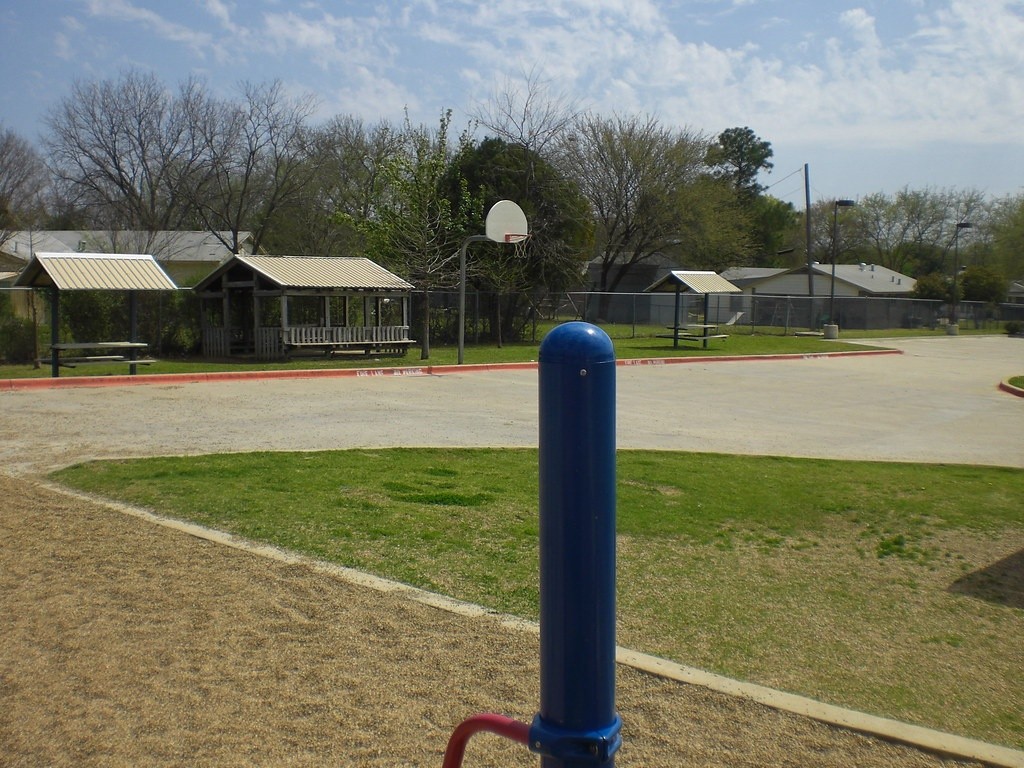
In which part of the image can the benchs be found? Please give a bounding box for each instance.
[684,335,729,340]
[65,360,156,367]
[34,355,123,361]
[655,333,690,336]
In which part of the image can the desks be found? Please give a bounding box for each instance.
[666,325,719,329]
[46,342,148,349]
